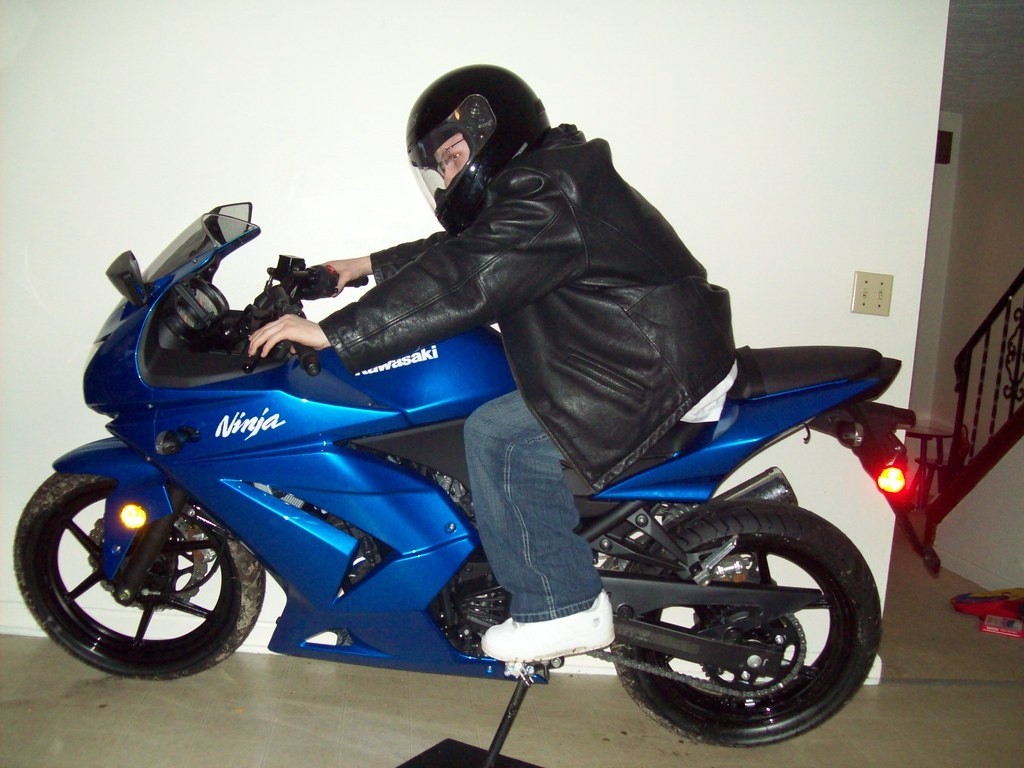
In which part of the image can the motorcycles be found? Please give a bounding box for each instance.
[13,200,915,768]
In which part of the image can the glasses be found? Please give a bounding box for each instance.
[435,138,464,179]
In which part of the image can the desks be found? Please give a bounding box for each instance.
[906,427,954,511]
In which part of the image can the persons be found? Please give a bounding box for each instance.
[248,66,739,664]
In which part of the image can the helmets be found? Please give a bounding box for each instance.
[406,65,550,237]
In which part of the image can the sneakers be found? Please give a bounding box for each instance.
[480,587,616,662]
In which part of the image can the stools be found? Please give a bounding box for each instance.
[913,458,947,504]
